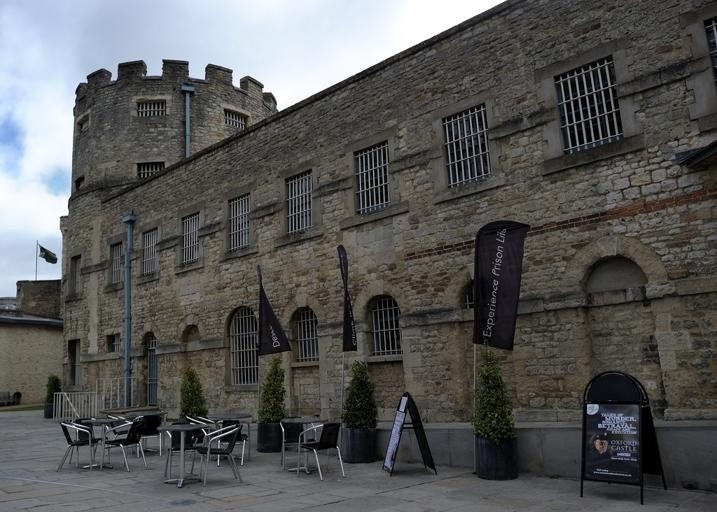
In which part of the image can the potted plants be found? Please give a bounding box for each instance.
[253,354,290,453]
[169,368,208,451]
[467,345,521,481]
[334,357,381,464]
[42,374,63,420]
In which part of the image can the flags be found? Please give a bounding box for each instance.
[38,246,57,264]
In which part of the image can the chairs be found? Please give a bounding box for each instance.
[53,404,349,489]
[0,391,21,406]
